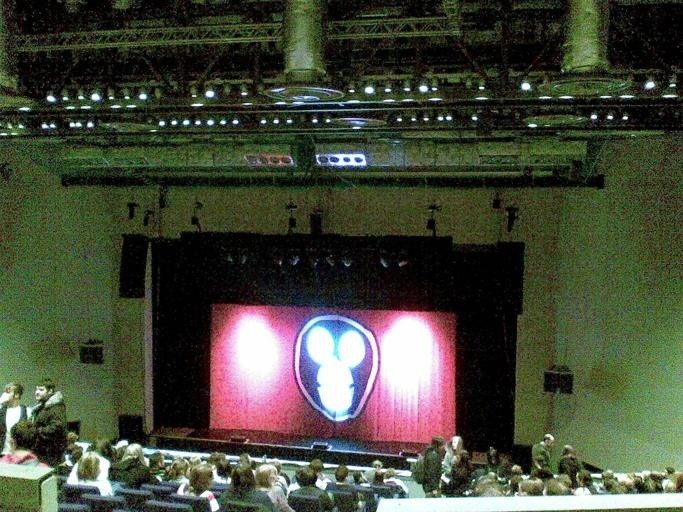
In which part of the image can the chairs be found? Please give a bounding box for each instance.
[1,435,681,512]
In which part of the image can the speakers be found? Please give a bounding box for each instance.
[80,343,103,363]
[116,232,149,297]
[119,414,144,442]
[543,371,573,393]
[509,444,533,473]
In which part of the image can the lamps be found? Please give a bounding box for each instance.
[1,68,683,136]
[125,184,519,277]
